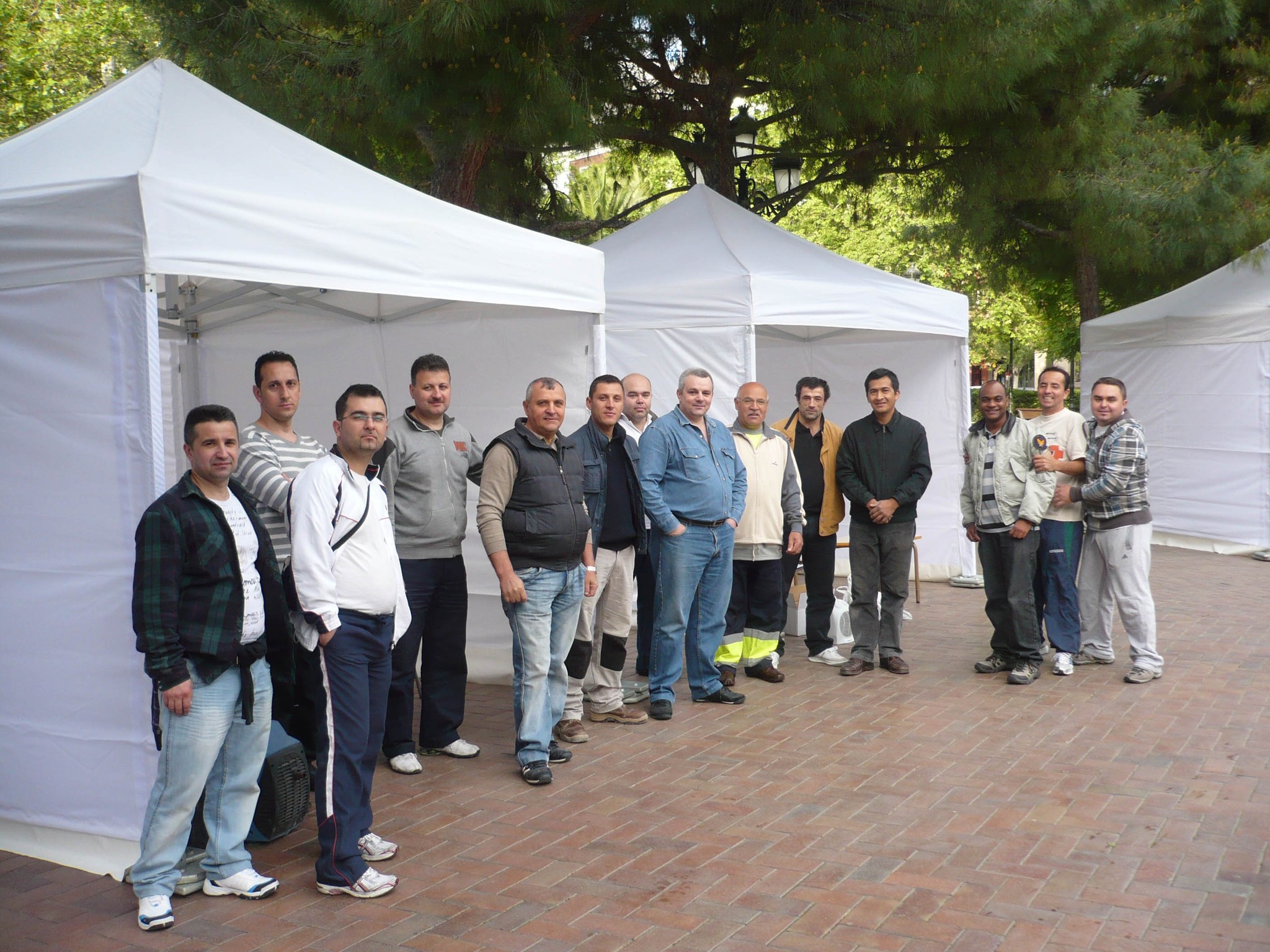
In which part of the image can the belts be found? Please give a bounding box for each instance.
[672,514,727,527]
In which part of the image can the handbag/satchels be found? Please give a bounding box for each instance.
[836,575,852,605]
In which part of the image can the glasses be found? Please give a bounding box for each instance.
[340,413,389,424]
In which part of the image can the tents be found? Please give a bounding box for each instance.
[2,56,608,883]
[1079,236,1270,560]
[590,168,986,589]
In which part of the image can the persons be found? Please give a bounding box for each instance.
[964,366,1082,675]
[834,368,932,676]
[713,381,808,689]
[372,352,487,774]
[127,405,280,932]
[617,373,656,677]
[478,377,598,784]
[638,366,747,720]
[960,380,1058,683]
[283,385,418,899]
[1052,377,1164,684]
[553,375,647,743]
[767,377,850,670]
[229,350,330,789]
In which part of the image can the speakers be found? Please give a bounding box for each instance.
[248,720,315,843]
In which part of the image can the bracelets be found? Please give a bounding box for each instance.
[585,566,597,574]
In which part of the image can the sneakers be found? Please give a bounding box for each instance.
[317,866,399,898]
[649,700,672,719]
[718,670,736,687]
[137,893,174,931]
[879,654,910,674]
[746,665,786,683]
[1124,666,1163,682]
[390,753,422,774]
[421,738,480,757]
[555,717,589,743]
[357,833,399,861]
[1007,659,1040,685]
[975,656,1013,673]
[1072,651,1115,665]
[521,760,553,784]
[548,740,572,763]
[840,659,874,676]
[202,866,281,900]
[1038,643,1049,656]
[695,685,746,704]
[590,704,647,724]
[1051,651,1073,676]
[808,646,848,666]
